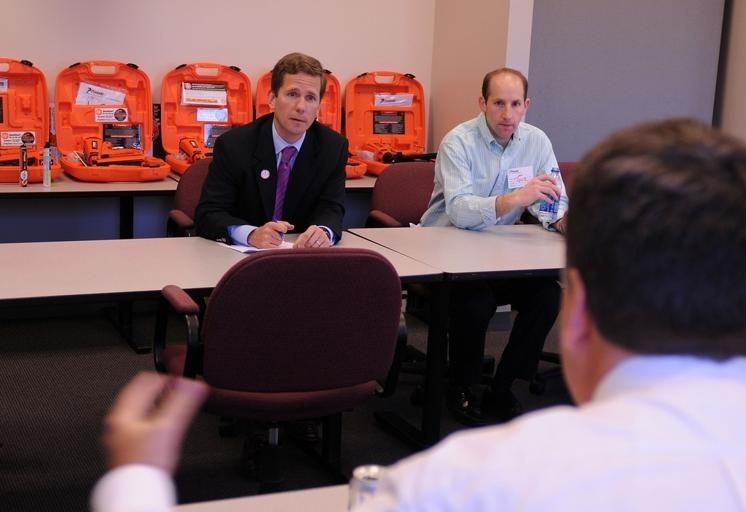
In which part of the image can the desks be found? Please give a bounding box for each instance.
[168,174,342,189]
[348,224,566,451]
[0,175,179,353]
[0,231,444,484]
[344,174,375,189]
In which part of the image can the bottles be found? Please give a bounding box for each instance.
[42,142,52,190]
[20,144,28,187]
[538,167,563,223]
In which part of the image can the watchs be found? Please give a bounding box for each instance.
[320,227,331,241]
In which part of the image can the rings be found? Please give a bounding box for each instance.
[316,241,321,247]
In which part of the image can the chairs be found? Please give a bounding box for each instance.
[151,247,408,496]
[164,156,213,237]
[369,162,545,420]
[558,160,577,197]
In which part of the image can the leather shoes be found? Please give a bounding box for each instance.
[484,385,521,416]
[447,386,475,423]
[293,420,322,461]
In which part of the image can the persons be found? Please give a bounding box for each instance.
[409,68,569,237]
[193,51,349,249]
[85,117,745,512]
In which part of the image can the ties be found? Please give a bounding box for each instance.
[275,147,296,237]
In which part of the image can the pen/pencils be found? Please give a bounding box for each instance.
[273,217,285,239]
